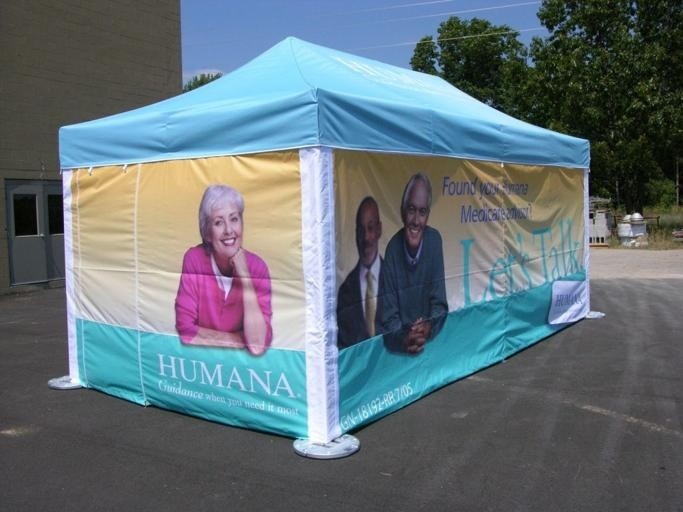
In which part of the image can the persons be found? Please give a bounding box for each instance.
[174,184,273,357]
[376,173,449,354]
[335,196,386,348]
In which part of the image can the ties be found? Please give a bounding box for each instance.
[363,271,378,337]
